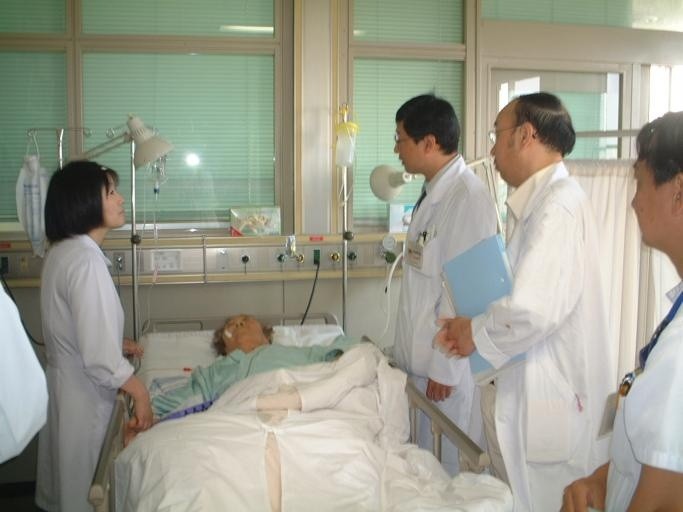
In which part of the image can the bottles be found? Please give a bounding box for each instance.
[336,121,358,166]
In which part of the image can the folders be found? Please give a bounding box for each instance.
[440,234,530,386]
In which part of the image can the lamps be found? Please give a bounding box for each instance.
[65,111,172,171]
[369,153,506,235]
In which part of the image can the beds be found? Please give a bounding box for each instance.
[88,310,491,512]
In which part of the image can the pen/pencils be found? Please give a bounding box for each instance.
[418,225,434,242]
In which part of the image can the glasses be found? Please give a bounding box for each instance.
[394,133,414,145]
[487,127,517,146]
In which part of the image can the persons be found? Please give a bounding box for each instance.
[120,302,381,449]
[387,89,504,479]
[0,278,51,465]
[30,159,153,511]
[556,106,683,512]
[430,91,623,512]
